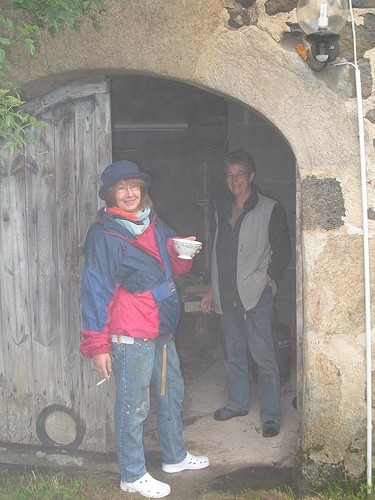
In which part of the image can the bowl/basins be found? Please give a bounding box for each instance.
[172,239,202,260]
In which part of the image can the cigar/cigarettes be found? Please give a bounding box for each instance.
[97,375,111,387]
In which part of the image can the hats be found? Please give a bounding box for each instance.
[98,160,151,200]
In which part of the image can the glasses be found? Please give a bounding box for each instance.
[116,184,140,192]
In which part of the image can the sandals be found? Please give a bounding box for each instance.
[213,407,247,420]
[262,421,279,436]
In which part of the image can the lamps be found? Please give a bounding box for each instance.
[296,0,348,73]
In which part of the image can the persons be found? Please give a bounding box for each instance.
[201,149,290,437]
[82,158,212,498]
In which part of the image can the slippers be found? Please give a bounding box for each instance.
[161,452,210,475]
[119,472,171,498]
